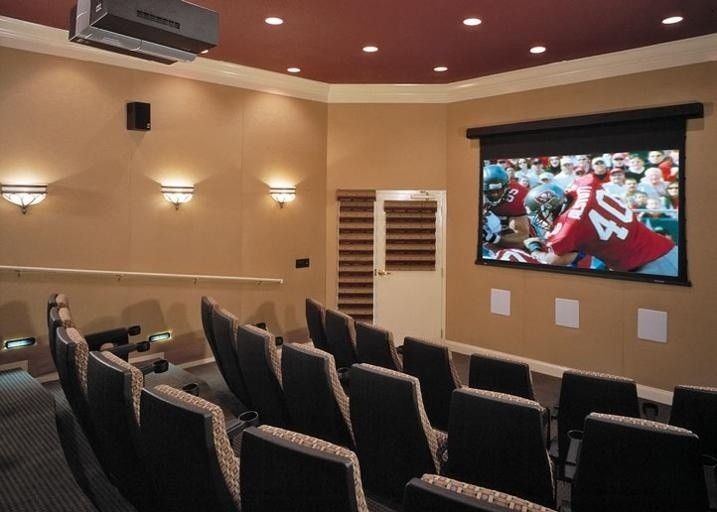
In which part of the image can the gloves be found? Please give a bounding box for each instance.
[524,237,541,252]
[482,223,496,243]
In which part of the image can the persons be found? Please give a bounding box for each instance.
[481,149,680,277]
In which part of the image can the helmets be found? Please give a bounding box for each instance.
[523,182,567,241]
[483,164,510,207]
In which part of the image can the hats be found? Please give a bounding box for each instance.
[560,157,572,165]
[592,158,604,166]
[612,153,625,160]
[609,168,624,174]
[533,159,543,164]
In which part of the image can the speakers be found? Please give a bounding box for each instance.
[127,101,151,131]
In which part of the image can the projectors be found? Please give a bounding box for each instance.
[68,0,221,66]
